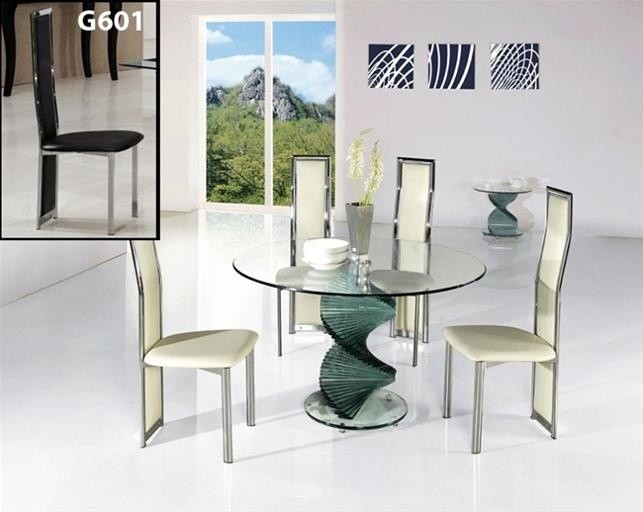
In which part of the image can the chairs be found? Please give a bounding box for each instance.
[277,154,338,357]
[129,239,258,464]
[390,156,435,367]
[33,8,145,235]
[442,186,573,453]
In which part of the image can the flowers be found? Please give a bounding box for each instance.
[346,126,381,205]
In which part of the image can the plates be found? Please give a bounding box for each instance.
[301,237,352,272]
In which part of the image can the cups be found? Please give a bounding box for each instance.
[355,259,372,292]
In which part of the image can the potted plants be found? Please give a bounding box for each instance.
[345,203,374,254]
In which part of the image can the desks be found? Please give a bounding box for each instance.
[474,184,533,238]
